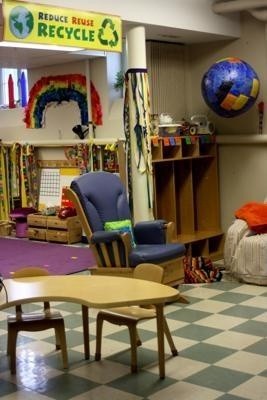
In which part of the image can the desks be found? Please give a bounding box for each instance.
[0,275,181,379]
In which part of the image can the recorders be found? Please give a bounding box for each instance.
[189,114,216,136]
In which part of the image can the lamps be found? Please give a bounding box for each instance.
[71,124,96,140]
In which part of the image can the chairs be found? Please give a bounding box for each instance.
[64,171,185,287]
[95,263,177,373]
[8,268,69,375]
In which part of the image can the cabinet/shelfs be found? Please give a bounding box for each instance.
[150,136,225,262]
[27,212,82,245]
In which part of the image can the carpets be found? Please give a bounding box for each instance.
[0,236,97,280]
